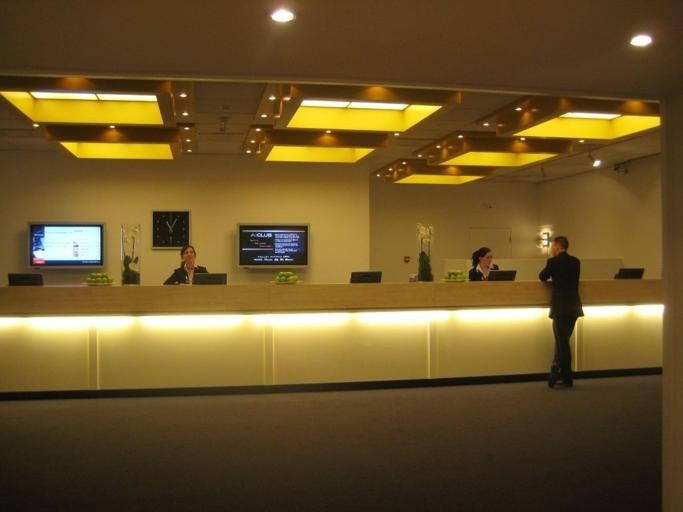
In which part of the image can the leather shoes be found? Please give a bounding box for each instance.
[558,381,572,387]
[549,365,560,388]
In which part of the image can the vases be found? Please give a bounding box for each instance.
[417,251,433,281]
[120,262,140,286]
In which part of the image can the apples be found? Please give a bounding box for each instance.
[86,273,113,283]
[444,269,466,279]
[276,272,299,282]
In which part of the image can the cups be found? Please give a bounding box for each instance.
[408,273,418,281]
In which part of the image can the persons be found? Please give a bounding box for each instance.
[538,236,584,387]
[163,244,209,285]
[469,247,499,281]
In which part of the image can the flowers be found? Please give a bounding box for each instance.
[121,221,141,266]
[414,221,434,257]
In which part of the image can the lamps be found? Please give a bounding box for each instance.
[474,94,663,139]
[409,129,579,168]
[367,155,497,186]
[0,73,196,130]
[44,121,198,161]
[253,81,462,134]
[537,231,551,248]
[242,123,389,166]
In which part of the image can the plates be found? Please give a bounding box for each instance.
[440,278,471,281]
[80,282,119,285]
[268,280,306,284]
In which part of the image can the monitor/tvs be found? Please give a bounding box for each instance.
[234,222,309,270]
[615,268,644,279]
[193,273,227,285]
[28,223,104,268]
[350,271,382,283]
[8,273,44,286]
[488,270,516,280]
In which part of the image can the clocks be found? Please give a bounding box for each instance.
[150,210,191,251]
